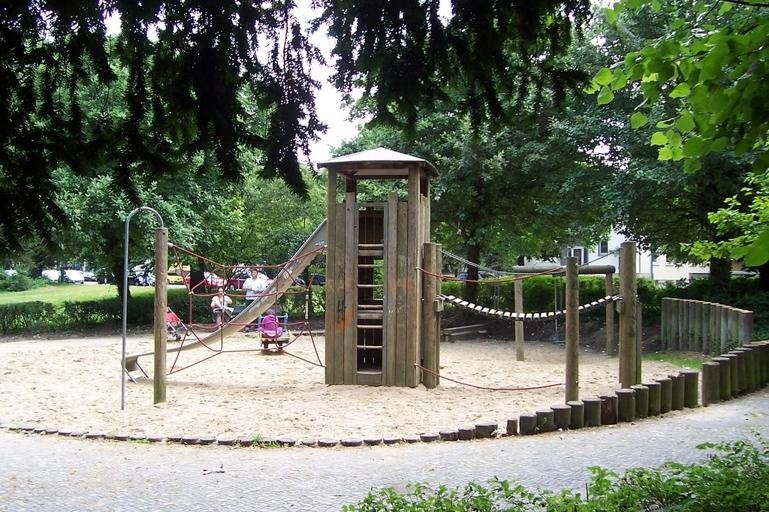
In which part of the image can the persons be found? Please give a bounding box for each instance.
[242,268,265,332]
[166,304,182,341]
[210,287,232,329]
[260,309,282,353]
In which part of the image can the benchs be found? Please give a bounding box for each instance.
[220,303,282,318]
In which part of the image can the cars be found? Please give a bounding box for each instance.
[3,267,115,285]
[125,257,326,291]
[455,271,483,283]
[441,272,455,281]
[480,272,496,278]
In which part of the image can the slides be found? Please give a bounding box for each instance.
[122,218,326,380]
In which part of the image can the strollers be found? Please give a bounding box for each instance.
[163,304,192,344]
